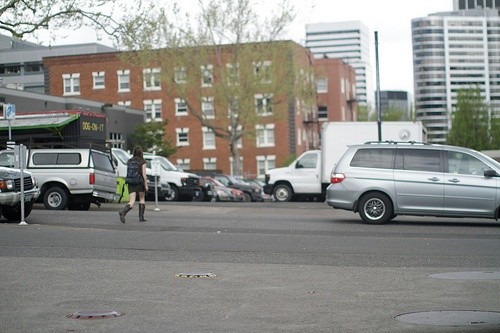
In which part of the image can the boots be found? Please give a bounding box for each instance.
[119,204,132,224]
[139,203,147,222]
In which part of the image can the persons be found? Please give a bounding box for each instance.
[118,146,148,224]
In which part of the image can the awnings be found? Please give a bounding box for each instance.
[0,113,80,130]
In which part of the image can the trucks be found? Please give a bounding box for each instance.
[263,122,427,201]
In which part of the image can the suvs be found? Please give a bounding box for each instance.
[0,166,40,223]
[130,155,201,201]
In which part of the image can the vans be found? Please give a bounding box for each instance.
[326,140,500,223]
[110,149,134,176]
[0,149,116,211]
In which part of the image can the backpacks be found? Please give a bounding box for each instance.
[124,159,146,185]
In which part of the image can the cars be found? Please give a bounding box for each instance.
[194,174,243,202]
[212,174,264,202]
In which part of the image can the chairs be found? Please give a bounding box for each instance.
[447,158,459,173]
